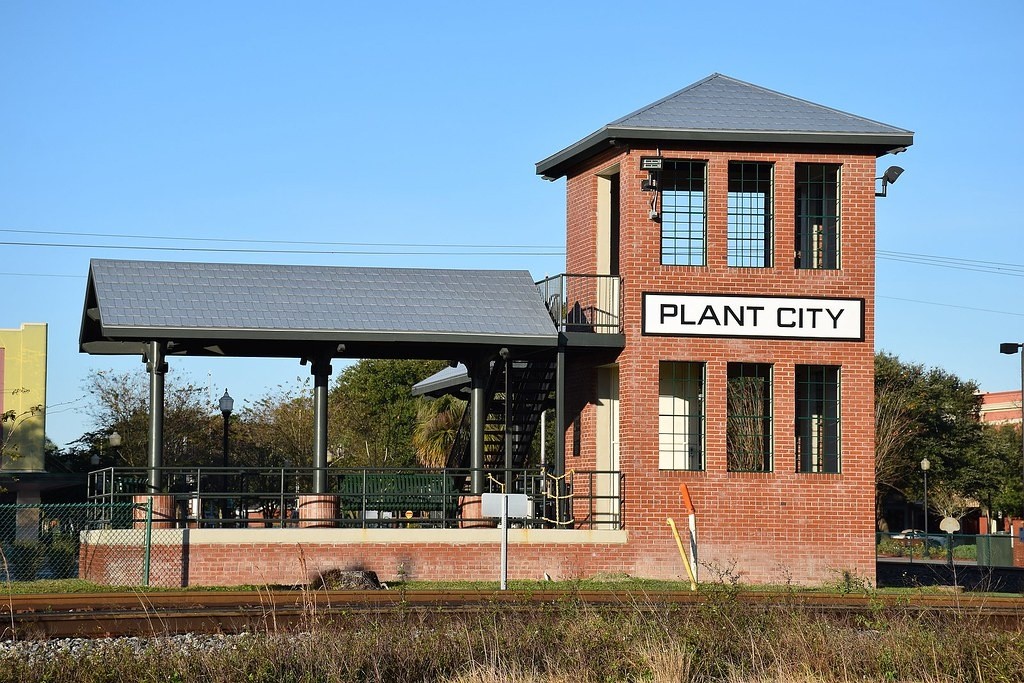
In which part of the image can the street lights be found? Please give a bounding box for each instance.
[219,387,234,528]
[110,432,121,502]
[91,454,99,471]
[920,456,931,556]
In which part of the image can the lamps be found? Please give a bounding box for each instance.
[639,155,663,191]
[875,166,905,197]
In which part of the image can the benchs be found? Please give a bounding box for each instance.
[336,472,460,528]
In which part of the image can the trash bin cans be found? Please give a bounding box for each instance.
[975,532,1014,566]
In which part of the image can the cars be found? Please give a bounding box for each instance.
[891,529,947,547]
